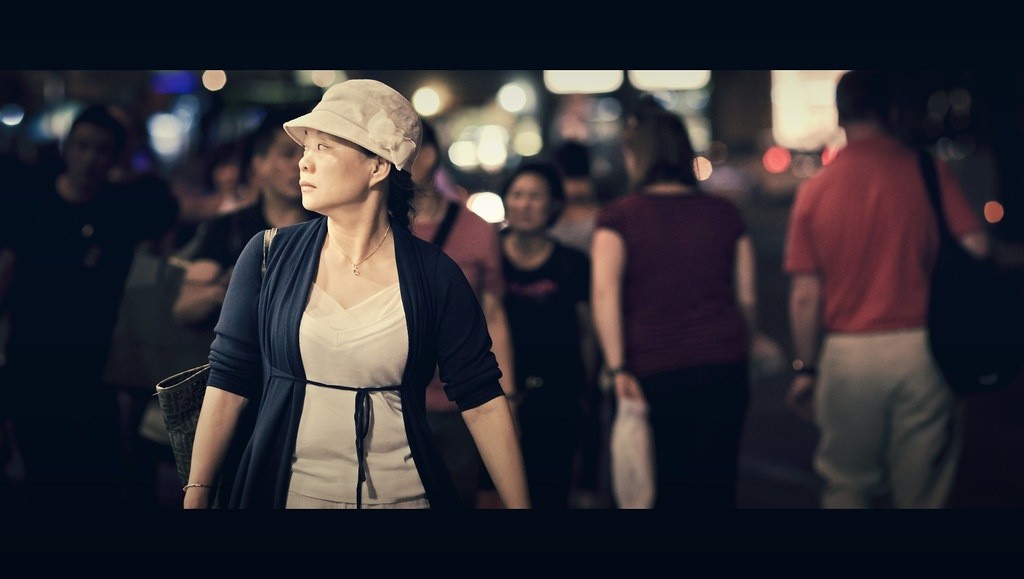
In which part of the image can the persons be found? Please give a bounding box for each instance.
[590,102,759,511]
[182,80,529,510]
[496,157,595,509]
[780,68,994,512]
[409,115,517,509]
[1,92,317,508]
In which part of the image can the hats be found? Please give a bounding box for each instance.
[283,79,425,175]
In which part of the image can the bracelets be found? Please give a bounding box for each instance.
[606,365,628,378]
[794,364,816,378]
[182,483,215,493]
[505,392,518,401]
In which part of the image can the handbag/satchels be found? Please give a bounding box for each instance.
[610,398,655,510]
[104,246,221,387]
[156,363,213,486]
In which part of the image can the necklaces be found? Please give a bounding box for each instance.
[325,223,391,276]
